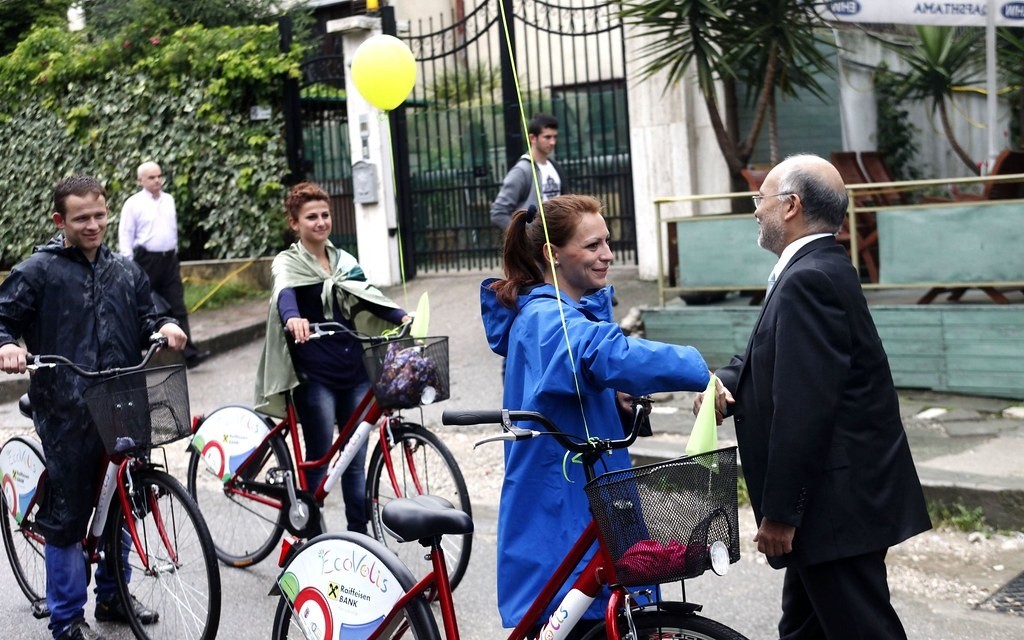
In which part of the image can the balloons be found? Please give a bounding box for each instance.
[351,34,418,110]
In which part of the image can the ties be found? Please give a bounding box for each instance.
[765,271,775,302]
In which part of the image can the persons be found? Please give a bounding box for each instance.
[271,183,414,537]
[479,193,710,640]
[115,161,214,370]
[0,174,189,640]
[489,113,568,233]
[691,150,937,640]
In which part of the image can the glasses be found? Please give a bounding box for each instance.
[752,191,796,209]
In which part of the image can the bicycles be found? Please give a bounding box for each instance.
[185,318,475,599]
[0,333,222,640]
[267,395,750,640]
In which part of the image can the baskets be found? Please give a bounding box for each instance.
[363,335,451,409]
[584,443,740,588]
[81,363,192,457]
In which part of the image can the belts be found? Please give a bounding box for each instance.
[147,249,177,257]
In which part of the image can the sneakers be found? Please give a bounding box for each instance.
[94,591,159,625]
[56,618,108,640]
